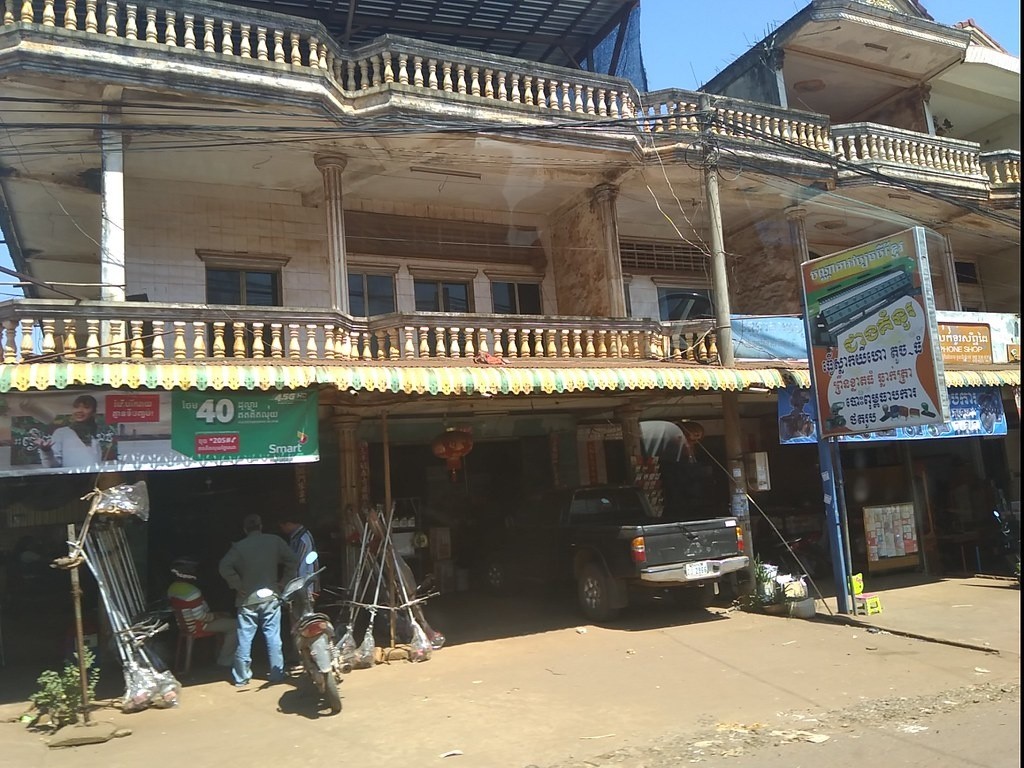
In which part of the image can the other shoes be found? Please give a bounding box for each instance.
[214,664,233,671]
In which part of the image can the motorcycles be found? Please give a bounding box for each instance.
[256,551,344,714]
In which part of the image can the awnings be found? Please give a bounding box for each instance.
[0,364,788,396]
[944,370,1021,388]
[780,369,812,390]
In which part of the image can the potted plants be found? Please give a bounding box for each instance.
[758,583,786,614]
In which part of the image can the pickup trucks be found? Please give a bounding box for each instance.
[484,482,750,620]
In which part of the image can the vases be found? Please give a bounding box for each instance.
[787,597,817,618]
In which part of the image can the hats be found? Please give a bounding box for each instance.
[243,513,262,528]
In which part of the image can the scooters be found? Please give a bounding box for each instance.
[992,510,1021,582]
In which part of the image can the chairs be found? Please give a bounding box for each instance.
[170,597,223,677]
[847,573,881,616]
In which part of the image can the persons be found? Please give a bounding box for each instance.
[32,395,102,468]
[219,513,299,687]
[275,511,320,673]
[167,555,238,669]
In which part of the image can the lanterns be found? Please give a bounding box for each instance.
[430,426,474,482]
[675,419,705,464]
